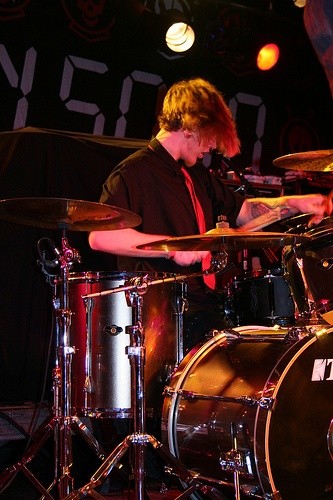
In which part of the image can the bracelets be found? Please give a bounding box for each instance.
[167,235,176,261]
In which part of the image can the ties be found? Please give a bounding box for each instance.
[181,165,216,291]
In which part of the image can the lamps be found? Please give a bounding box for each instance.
[156,8,197,55]
[245,27,279,71]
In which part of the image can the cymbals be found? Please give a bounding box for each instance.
[0,197,143,230]
[272,150,333,172]
[135,229,312,251]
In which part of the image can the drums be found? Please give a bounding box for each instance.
[159,324,333,500]
[53,267,189,422]
[223,266,310,325]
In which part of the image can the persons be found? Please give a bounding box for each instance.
[87,77,333,491]
